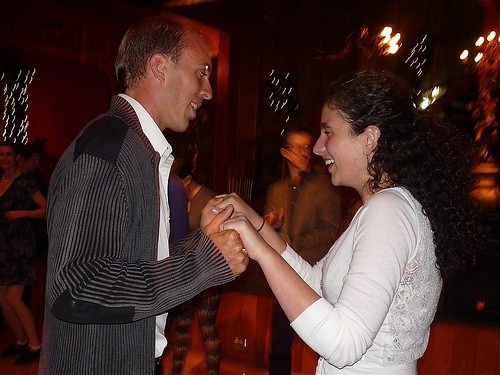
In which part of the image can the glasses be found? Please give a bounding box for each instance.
[285,144,312,150]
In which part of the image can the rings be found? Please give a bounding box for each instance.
[241,249,248,255]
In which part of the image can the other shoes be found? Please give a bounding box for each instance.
[0,341,27,360]
[14,347,42,366]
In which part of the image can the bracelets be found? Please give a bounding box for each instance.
[257,219,265,232]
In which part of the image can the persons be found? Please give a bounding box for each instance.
[38,17,250,375]
[263,131,342,375]
[169,164,222,375]
[213,70,491,374]
[0,139,49,365]
